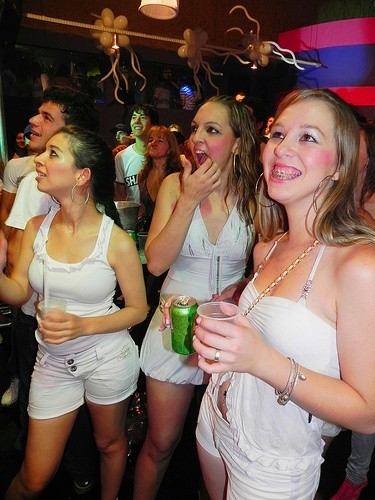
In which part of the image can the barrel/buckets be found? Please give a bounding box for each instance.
[95,201,142,250]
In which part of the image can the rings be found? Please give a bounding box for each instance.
[213,349,221,362]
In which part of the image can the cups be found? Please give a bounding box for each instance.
[137,232,148,256]
[37,297,67,341]
[197,300,241,366]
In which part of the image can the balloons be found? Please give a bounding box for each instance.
[176,27,209,70]
[88,7,130,56]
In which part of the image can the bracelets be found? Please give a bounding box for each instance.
[277,357,307,406]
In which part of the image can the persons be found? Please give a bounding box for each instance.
[31,65,204,110]
[134,94,284,500]
[235,97,275,195]
[0,85,99,452]
[330,124,375,500]
[158,87,375,500]
[241,31,273,68]
[0,123,149,500]
[107,103,196,250]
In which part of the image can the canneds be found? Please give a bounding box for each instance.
[169,295,200,355]
[126,229,137,248]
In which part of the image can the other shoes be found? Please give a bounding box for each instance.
[1,378,19,405]
[14,428,26,450]
[72,461,97,494]
[331,478,368,500]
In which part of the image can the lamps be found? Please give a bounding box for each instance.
[138,0,179,20]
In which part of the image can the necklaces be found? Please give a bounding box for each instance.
[209,189,234,213]
